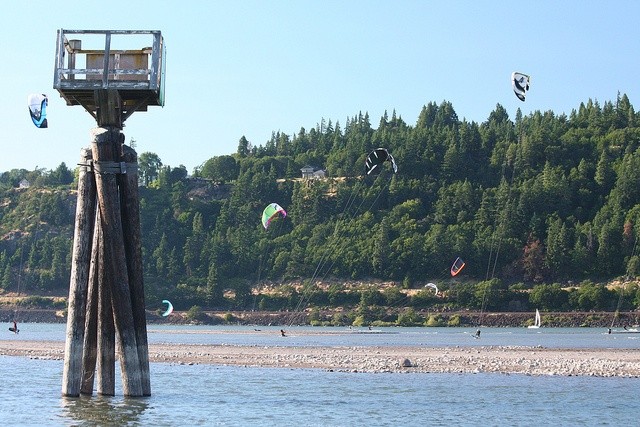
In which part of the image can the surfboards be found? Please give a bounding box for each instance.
[8,327,20,332]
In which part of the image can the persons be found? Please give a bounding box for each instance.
[369,324,372,330]
[623,320,629,330]
[605,328,618,334]
[472,329,481,339]
[281,328,286,336]
[13,320,17,333]
